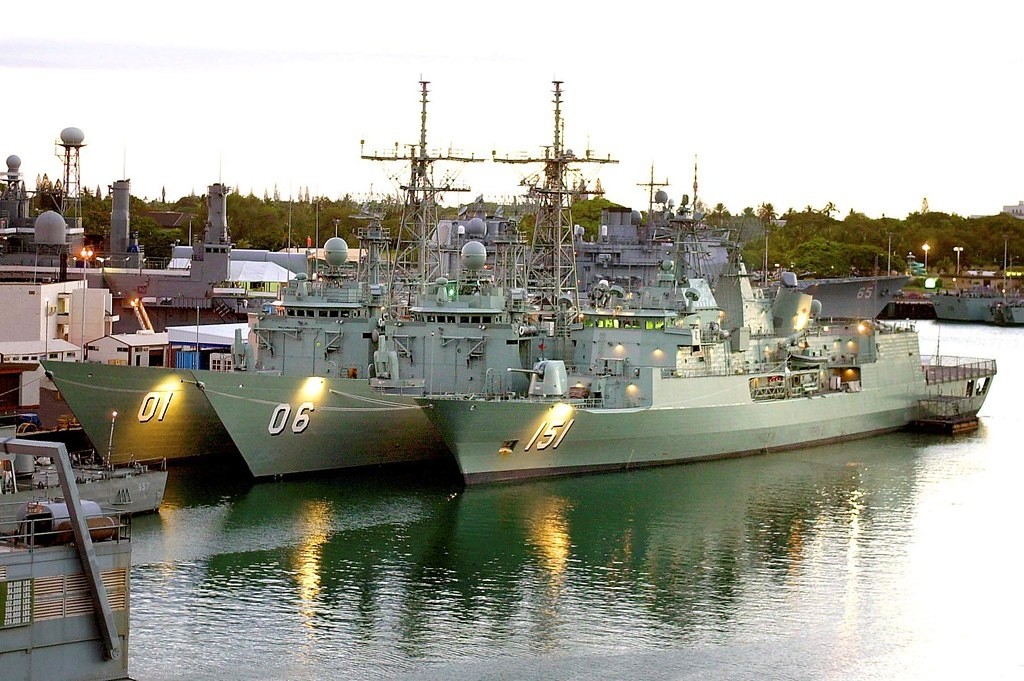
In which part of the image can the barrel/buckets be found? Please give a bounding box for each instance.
[340,368,349,378]
[349,368,357,379]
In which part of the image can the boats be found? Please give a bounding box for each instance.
[1,412,167,533]
[37,77,998,488]
[928,285,1024,327]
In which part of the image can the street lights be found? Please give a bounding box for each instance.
[922,245,930,270]
[954,247,963,277]
[80,250,93,363]
